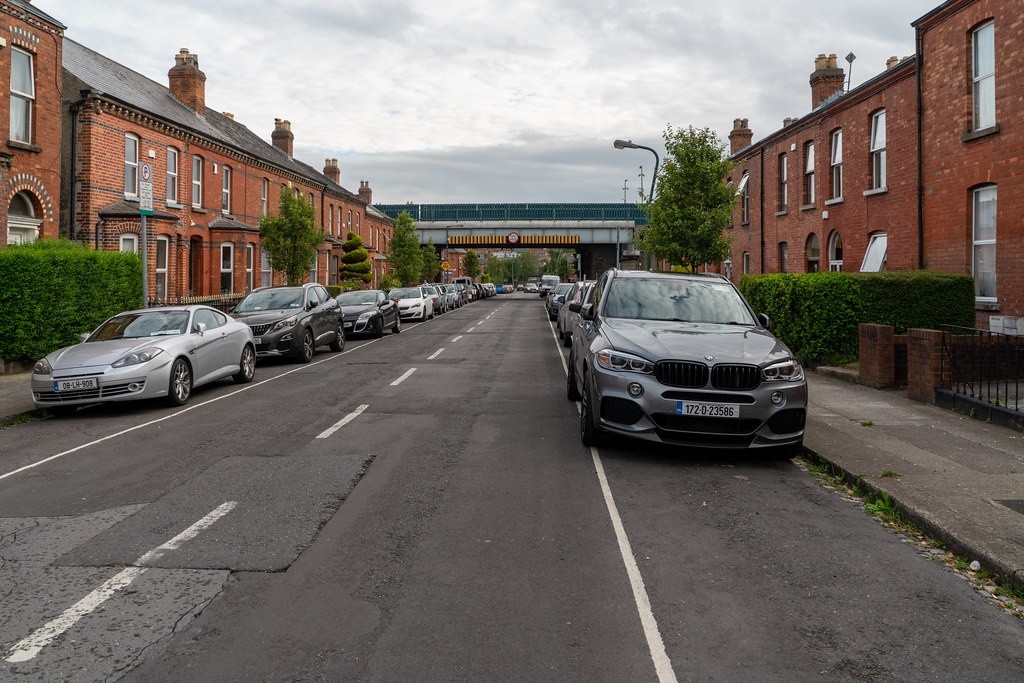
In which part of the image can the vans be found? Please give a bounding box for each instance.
[540,275,561,299]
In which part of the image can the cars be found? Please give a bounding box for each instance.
[335,289,402,338]
[385,287,434,322]
[30,305,258,417]
[525,282,540,293]
[505,287,513,293]
[419,277,498,315]
[547,283,578,321]
[496,284,506,294]
[557,279,597,347]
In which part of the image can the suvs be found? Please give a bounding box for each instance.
[226,282,345,363]
[566,267,809,460]
[517,284,525,291]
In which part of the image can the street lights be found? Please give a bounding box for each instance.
[512,261,515,283]
[613,139,660,203]
